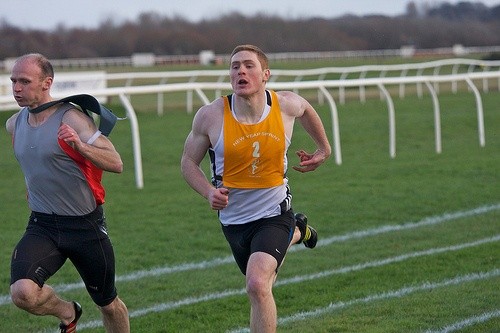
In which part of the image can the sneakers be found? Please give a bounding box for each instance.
[59,300,82,333]
[295,212,318,248]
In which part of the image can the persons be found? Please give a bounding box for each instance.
[6,54,139,332]
[181,45,332,332]
